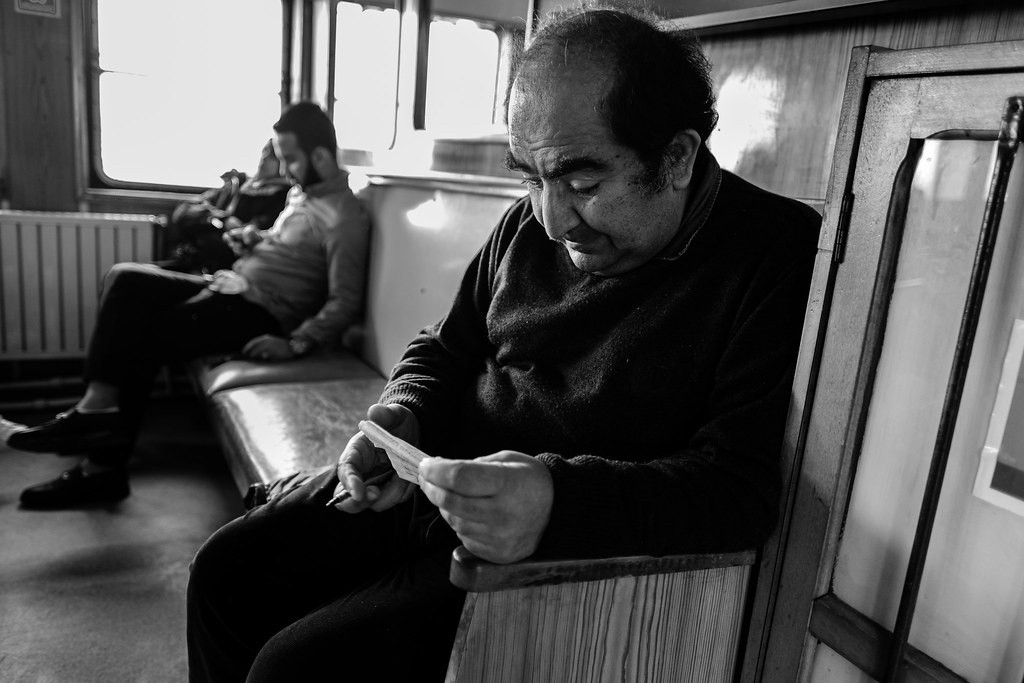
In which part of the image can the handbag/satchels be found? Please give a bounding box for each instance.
[172,176,240,224]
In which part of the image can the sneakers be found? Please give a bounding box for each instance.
[21,457,129,509]
[7,402,135,451]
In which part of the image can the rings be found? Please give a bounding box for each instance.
[262,351,268,358]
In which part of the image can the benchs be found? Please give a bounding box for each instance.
[183,173,759,683]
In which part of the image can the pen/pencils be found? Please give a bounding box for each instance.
[325,468,395,507]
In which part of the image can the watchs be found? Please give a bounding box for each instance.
[288,338,307,357]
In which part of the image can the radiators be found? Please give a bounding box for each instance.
[0,211,166,426]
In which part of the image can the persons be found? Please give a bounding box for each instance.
[7,101,370,513]
[188,7,822,682]
